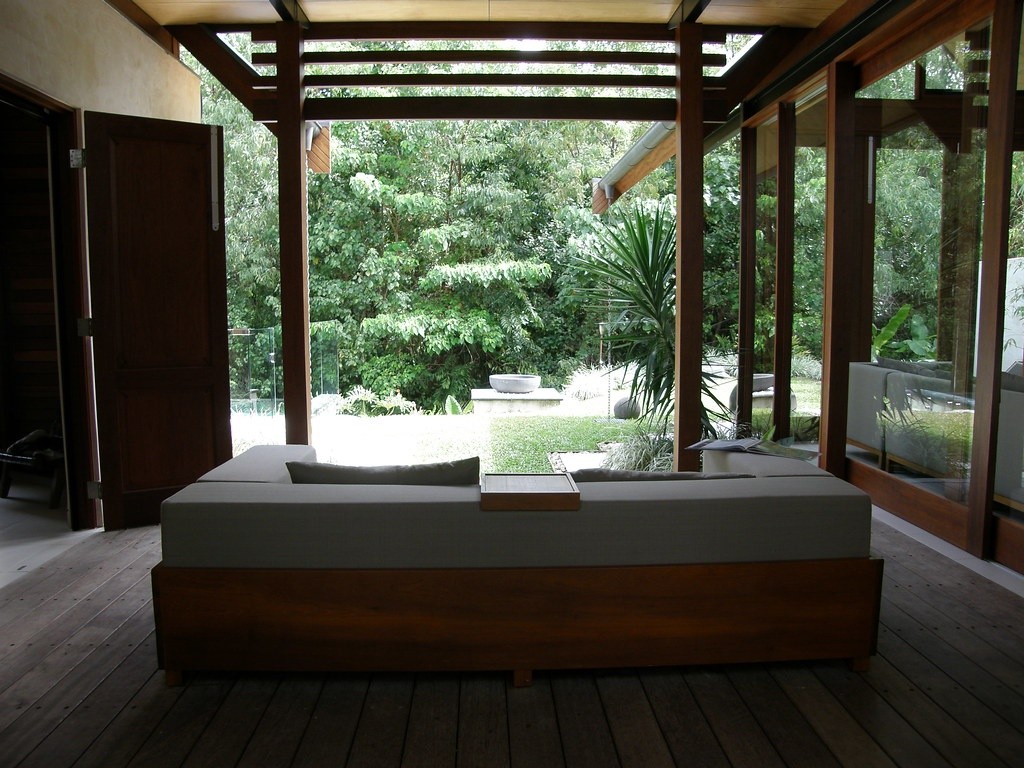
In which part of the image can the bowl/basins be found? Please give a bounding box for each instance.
[488,373,542,393]
[751,373,775,392]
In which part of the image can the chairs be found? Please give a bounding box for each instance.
[0,419,66,509]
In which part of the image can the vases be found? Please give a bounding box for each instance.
[753,372,774,392]
[489,374,541,392]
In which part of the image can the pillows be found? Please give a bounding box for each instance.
[286,456,480,483]
[874,354,934,378]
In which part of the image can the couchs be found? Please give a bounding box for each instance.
[151,442,884,688]
[850,356,1024,515]
